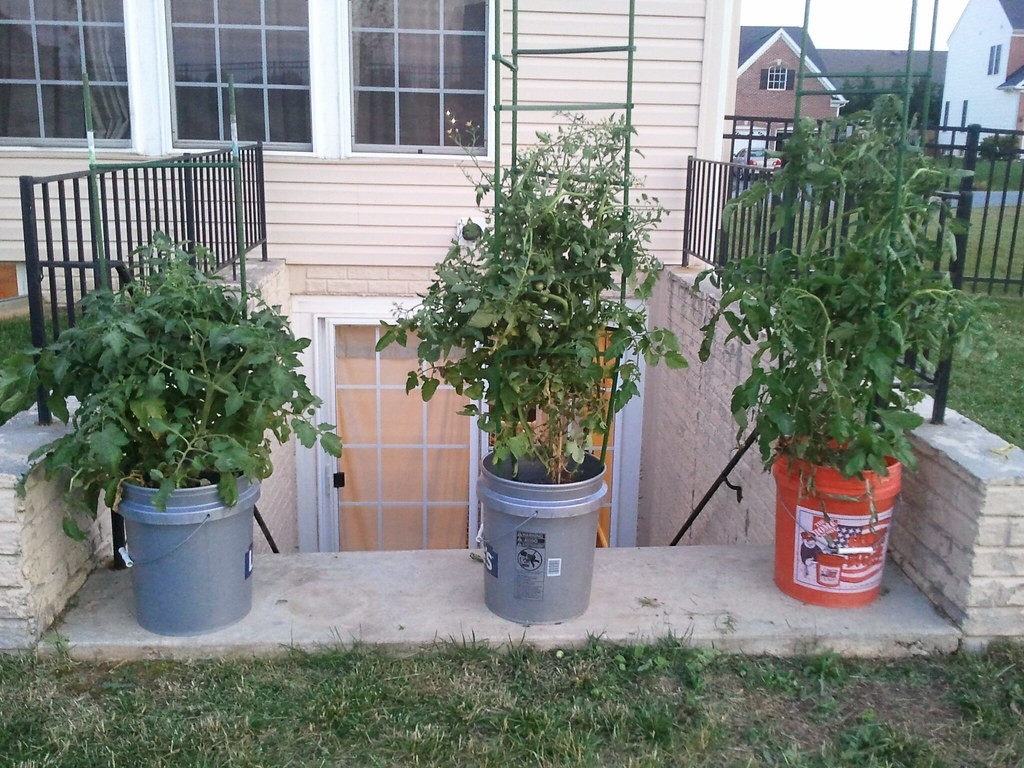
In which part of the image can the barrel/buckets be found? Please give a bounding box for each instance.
[476,446,608,625]
[118,474,261,637]
[773,436,902,607]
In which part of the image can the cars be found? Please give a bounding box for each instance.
[733,147,782,181]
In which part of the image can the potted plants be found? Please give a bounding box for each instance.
[690,93,1007,608]
[374,102,689,626]
[0,229,343,637]
